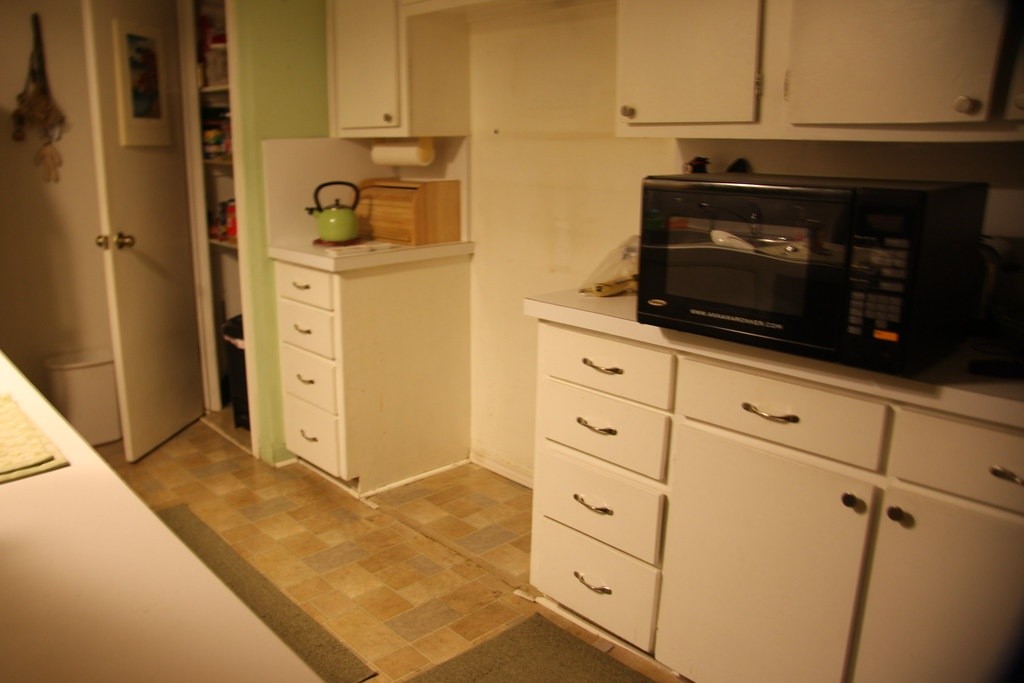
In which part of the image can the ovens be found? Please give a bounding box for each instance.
[628,173,991,384]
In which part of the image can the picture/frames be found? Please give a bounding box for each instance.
[110,18,173,147]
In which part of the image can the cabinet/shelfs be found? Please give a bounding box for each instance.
[613,0,1024,142]
[522,293,1024,683]
[177,0,330,460]
[266,233,475,499]
[324,0,470,140]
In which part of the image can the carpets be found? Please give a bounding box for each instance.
[400,612,660,682]
[153,503,379,683]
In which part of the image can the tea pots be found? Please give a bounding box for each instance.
[304,181,361,245]
